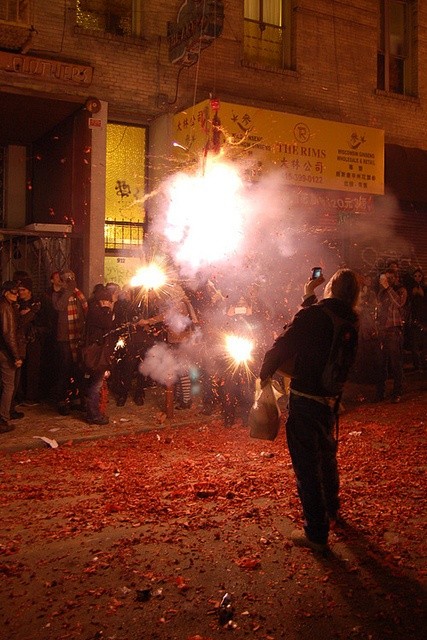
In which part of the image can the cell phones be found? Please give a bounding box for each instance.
[380,274,386,279]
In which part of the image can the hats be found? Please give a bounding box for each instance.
[60,270,75,281]
[17,278,32,290]
[0,281,17,296]
[94,286,112,301]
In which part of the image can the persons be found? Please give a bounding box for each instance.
[13,270,27,282]
[353,274,375,385]
[116,286,144,407]
[51,269,89,416]
[201,276,228,417]
[259,267,358,551]
[219,295,265,427]
[137,285,198,410]
[106,282,123,394]
[0,281,25,433]
[377,271,409,404]
[407,268,427,373]
[82,283,111,423]
[17,278,52,407]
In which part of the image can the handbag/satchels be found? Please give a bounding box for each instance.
[79,313,112,372]
[248,379,283,441]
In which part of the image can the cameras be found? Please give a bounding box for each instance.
[312,267,322,280]
[131,314,141,323]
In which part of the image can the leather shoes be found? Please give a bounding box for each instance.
[10,411,24,419]
[175,402,191,410]
[117,392,126,406]
[135,394,143,405]
[0,423,14,433]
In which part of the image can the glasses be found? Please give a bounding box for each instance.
[9,290,18,294]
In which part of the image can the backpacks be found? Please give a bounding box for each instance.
[313,302,361,398]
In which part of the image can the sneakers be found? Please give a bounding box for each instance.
[291,528,329,553]
[86,417,107,424]
